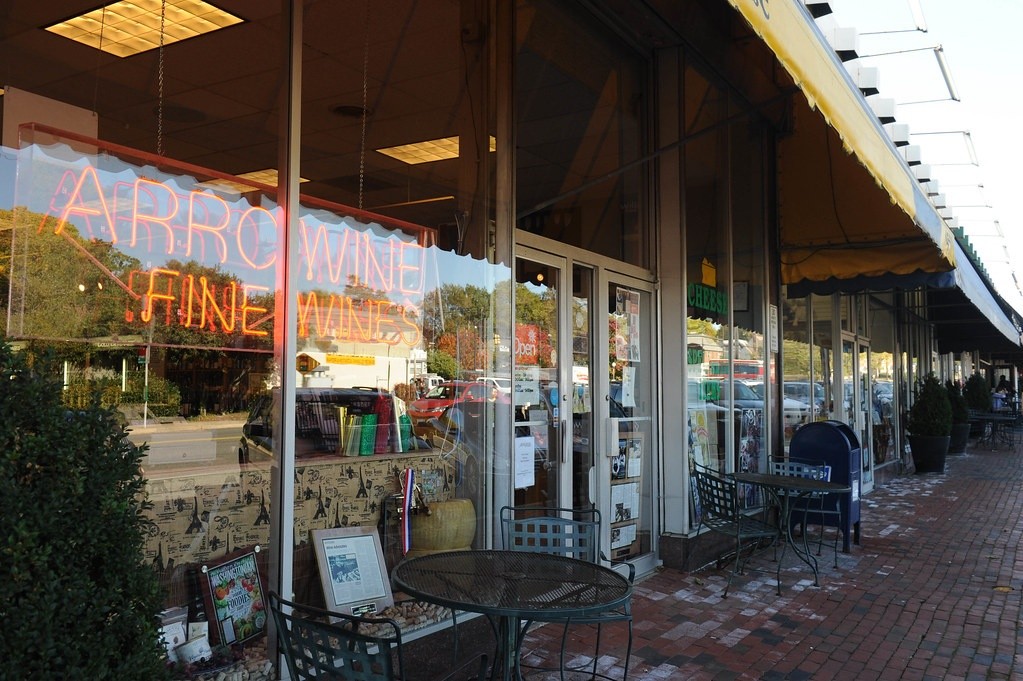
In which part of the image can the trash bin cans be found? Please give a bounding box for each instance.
[992,392,1006,408]
[785,419,862,553]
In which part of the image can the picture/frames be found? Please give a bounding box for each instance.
[311,526,394,624]
[198,541,268,650]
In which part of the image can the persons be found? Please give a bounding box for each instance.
[995,374,1017,395]
[1018,373,1023,411]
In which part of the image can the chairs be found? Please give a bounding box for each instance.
[678,459,788,601]
[770,457,827,554]
[499,506,635,681]
[266,589,488,681]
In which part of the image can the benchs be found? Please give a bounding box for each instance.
[728,473,853,587]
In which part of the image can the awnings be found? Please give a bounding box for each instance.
[726,0,1022,349]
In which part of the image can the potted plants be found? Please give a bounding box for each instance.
[964,371,994,438]
[906,372,971,472]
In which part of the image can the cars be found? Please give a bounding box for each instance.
[239,376,894,495]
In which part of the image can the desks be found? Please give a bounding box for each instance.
[975,414,1016,452]
[392,548,631,681]
